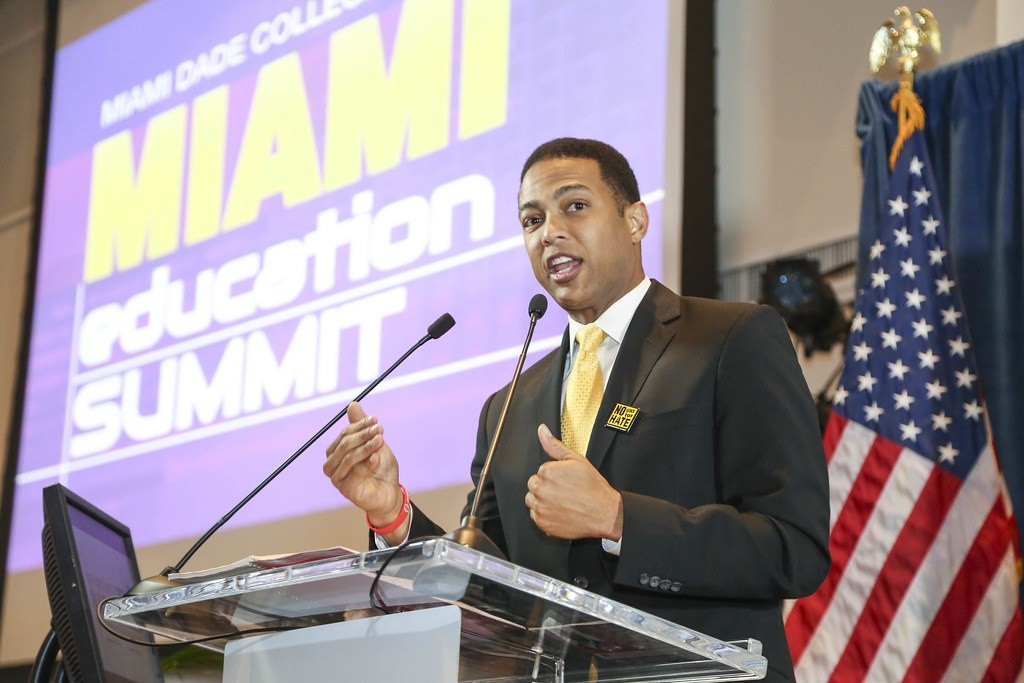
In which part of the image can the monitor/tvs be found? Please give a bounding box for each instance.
[40,483,164,683]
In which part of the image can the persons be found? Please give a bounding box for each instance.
[323,137,831,683]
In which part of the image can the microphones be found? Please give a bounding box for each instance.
[442,294,548,562]
[120,313,455,598]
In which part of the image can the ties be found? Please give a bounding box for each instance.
[559,324,610,459]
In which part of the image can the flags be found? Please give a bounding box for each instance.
[780,40,1024,683]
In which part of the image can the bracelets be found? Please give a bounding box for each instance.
[365,484,410,535]
